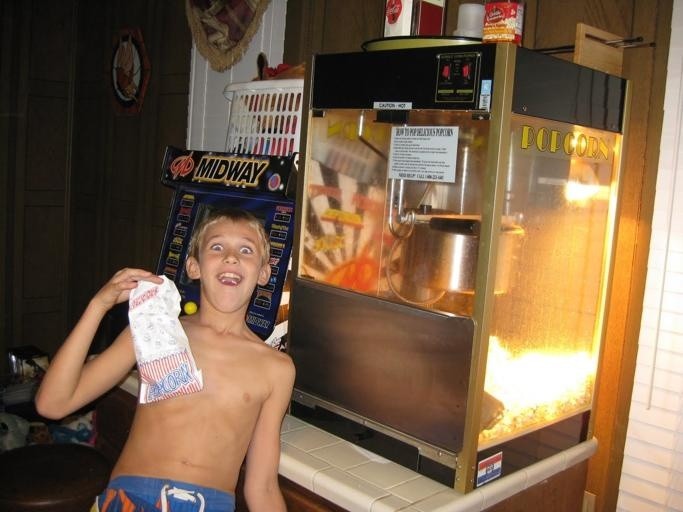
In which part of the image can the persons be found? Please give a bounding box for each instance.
[34,209,297,512]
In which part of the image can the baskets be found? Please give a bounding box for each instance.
[222,79,304,157]
